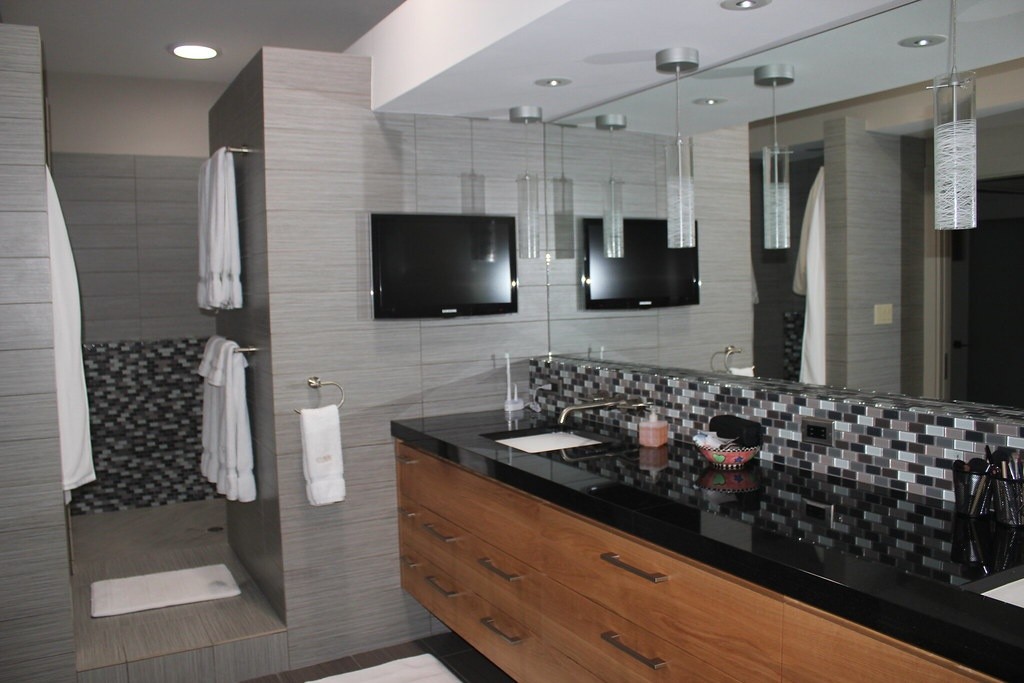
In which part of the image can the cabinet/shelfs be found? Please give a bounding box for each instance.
[395,438,1009,683]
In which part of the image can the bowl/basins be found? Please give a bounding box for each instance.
[695,442,761,472]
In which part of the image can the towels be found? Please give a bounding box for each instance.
[196,333,258,504]
[298,403,347,507]
[195,145,246,316]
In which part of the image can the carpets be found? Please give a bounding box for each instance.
[304,653,463,683]
[90,563,241,617]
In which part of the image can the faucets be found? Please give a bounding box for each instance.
[557,396,626,425]
[559,449,619,463]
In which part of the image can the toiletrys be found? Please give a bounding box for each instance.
[639,448,669,478]
[638,409,669,448]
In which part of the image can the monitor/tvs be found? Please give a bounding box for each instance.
[370,212,518,319]
[581,217,699,310]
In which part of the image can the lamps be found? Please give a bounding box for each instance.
[934,0,977,230]
[655,47,699,248]
[596,114,626,261]
[754,63,794,252]
[510,106,542,259]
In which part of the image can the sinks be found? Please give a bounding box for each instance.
[979,576,1024,609]
[479,430,605,454]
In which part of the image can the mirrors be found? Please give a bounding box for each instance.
[544,0,1024,409]
